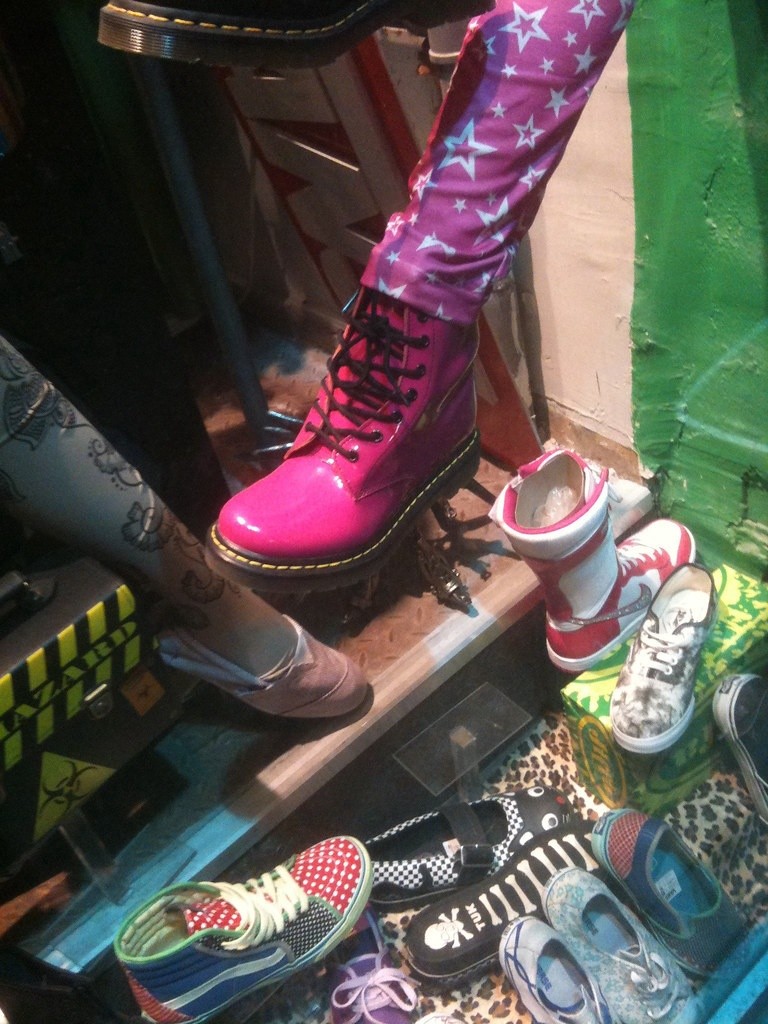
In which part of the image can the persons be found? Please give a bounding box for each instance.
[204,2,638,595]
[1,337,369,720]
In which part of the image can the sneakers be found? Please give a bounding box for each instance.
[114,835,373,1024]
[717,674,768,827]
[611,563,716,754]
[542,866,699,1024]
[151,604,369,720]
[365,781,598,989]
[499,913,616,1024]
[591,806,747,983]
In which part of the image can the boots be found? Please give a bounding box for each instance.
[493,445,695,674]
[209,287,482,575]
